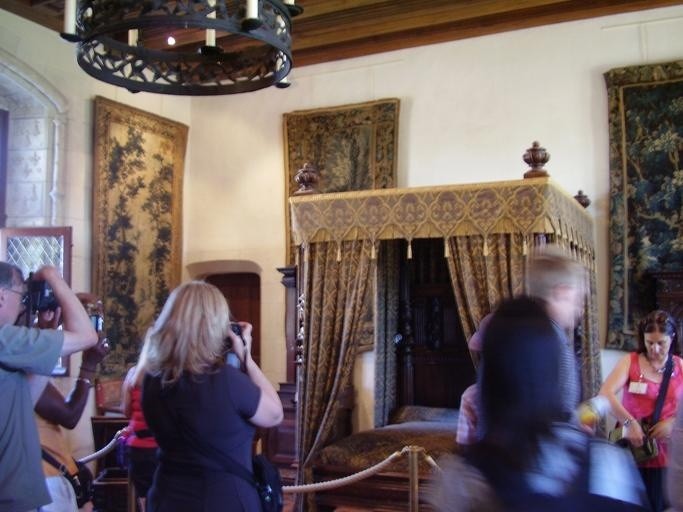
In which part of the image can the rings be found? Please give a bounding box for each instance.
[102,342,108,347]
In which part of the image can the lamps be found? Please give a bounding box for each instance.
[60,0,303,97]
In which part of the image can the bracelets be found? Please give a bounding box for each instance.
[74,375,92,387]
[78,365,97,373]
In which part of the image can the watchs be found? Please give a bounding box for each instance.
[622,416,636,429]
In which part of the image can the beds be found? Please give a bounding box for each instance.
[304,403,463,511]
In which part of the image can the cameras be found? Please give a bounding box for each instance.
[86,313,104,333]
[25,279,55,313]
[222,321,241,349]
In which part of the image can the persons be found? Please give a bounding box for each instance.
[68,455,98,511]
[465,282,526,350]
[27,293,108,511]
[597,309,683,511]
[136,280,284,511]
[423,297,650,512]
[453,377,482,448]
[116,326,159,512]
[527,245,590,433]
[0,263,98,511]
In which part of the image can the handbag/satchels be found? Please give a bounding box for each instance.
[609,422,659,463]
[253,454,284,512]
[71,462,94,509]
